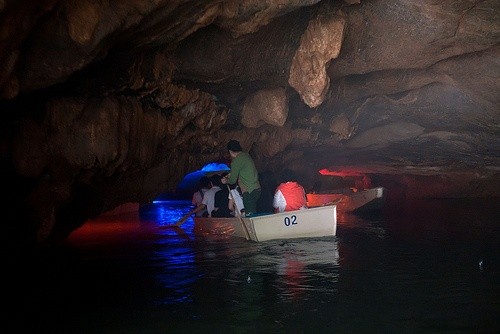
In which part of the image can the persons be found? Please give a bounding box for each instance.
[192,173,245,217]
[272,168,307,213]
[220,140,262,216]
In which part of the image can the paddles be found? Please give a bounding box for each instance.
[227,185,251,241]
[174,211,193,226]
